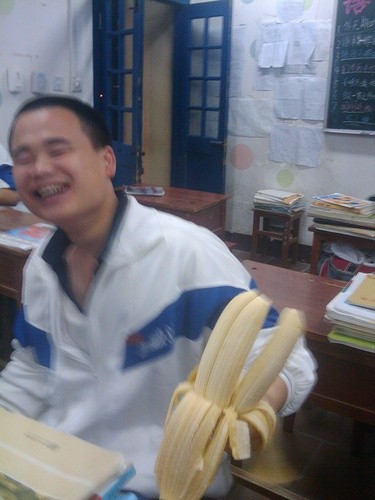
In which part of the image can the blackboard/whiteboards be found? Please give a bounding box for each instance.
[323,0,375,136]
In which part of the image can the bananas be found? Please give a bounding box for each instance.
[153,290,303,500]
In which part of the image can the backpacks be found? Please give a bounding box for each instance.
[317,240,375,281]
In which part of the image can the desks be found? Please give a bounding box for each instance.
[116,182,233,243]
[308,225,375,276]
[0,206,57,309]
[241,260,375,427]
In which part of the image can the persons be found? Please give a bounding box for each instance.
[0,142,20,207]
[0,94,317,500]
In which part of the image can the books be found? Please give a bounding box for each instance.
[253,189,305,211]
[1,410,139,499]
[123,184,164,196]
[0,222,56,253]
[325,269,374,356]
[310,190,375,239]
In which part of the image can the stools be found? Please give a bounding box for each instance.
[250,208,305,269]
